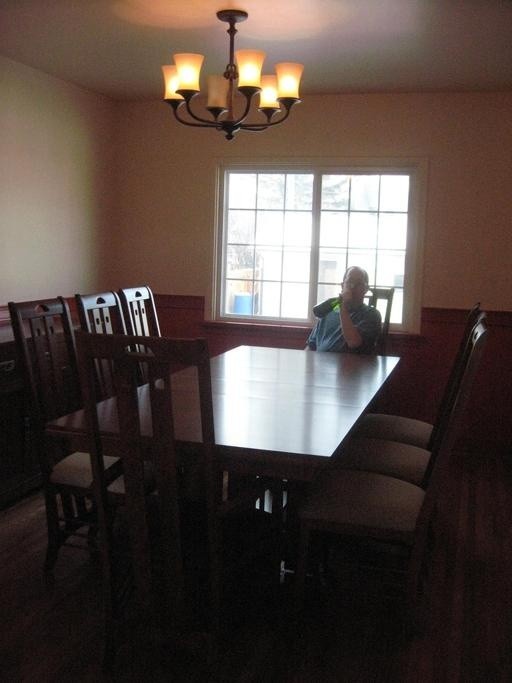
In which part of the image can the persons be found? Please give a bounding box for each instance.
[302,265,379,355]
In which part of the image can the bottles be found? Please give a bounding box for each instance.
[312,295,343,319]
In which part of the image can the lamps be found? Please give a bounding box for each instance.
[158,6,305,141]
